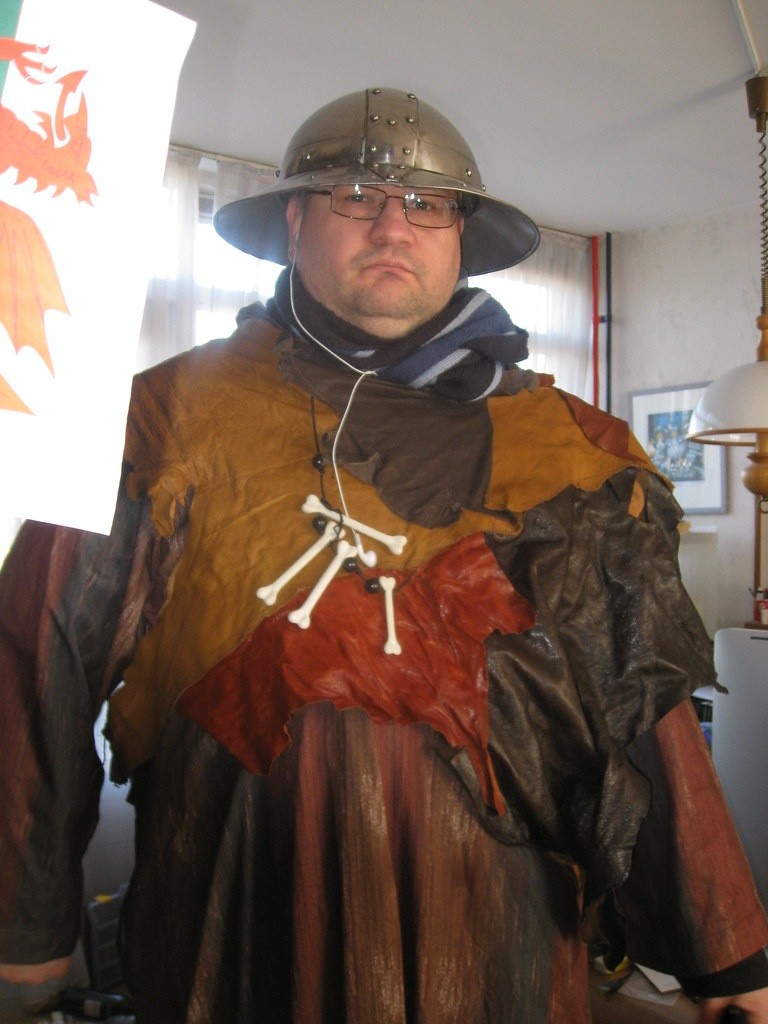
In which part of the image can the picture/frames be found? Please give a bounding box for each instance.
[625,380,728,514]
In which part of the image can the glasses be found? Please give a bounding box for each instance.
[303,183,471,229]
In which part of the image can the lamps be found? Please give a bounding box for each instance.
[683,77,768,512]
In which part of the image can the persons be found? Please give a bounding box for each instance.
[0,88,768,1024]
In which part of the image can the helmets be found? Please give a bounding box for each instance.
[216,90,541,275]
